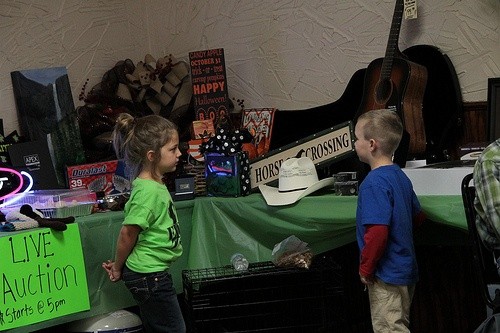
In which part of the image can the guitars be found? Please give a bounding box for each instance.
[352,0,430,166]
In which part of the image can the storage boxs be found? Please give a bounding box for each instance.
[67,159,118,194]
[4,188,96,219]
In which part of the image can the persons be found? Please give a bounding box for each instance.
[473,137,500,276]
[354,109,425,333]
[101,113,187,333]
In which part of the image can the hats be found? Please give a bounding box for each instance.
[258,157,335,206]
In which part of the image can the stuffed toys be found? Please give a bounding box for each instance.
[0,204,75,232]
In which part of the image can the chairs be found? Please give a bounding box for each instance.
[462,173,500,333]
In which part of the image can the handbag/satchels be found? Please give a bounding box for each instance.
[205,151,251,197]
[8,133,62,193]
